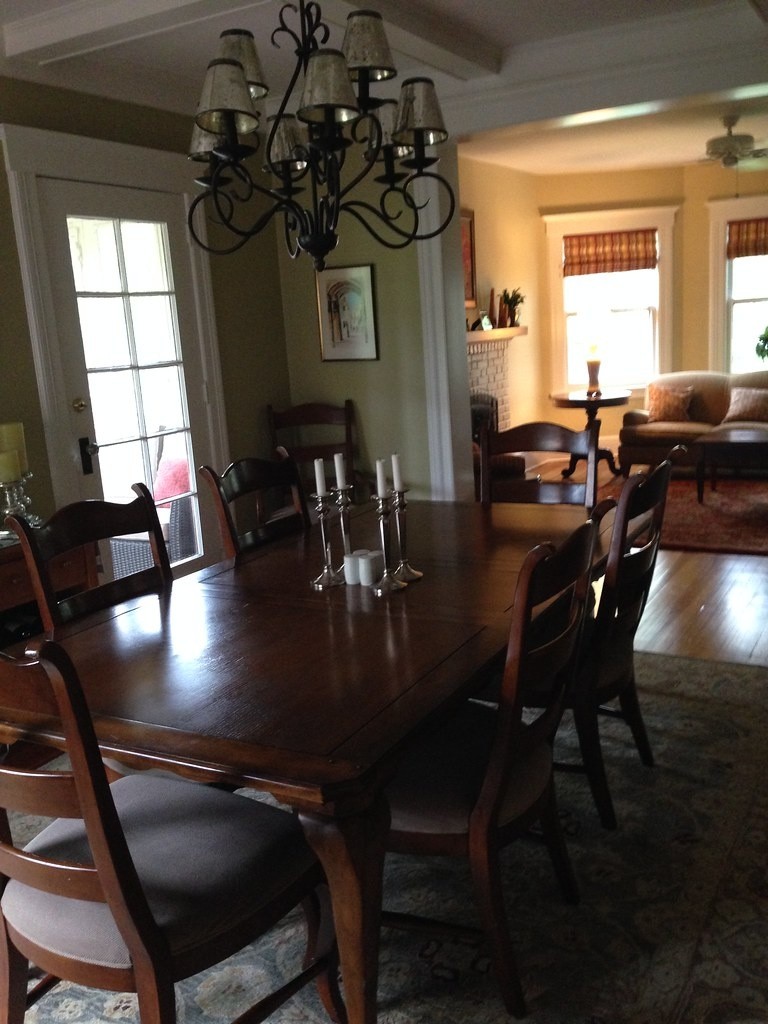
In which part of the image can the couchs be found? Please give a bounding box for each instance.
[618,371,768,489]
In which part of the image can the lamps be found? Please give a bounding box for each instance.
[187,1,457,271]
[704,115,754,166]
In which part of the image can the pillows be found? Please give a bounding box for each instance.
[720,386,768,425]
[648,383,693,422]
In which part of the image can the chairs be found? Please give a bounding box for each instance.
[1,394,670,1024]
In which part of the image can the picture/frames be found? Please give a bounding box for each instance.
[460,209,478,310]
[314,263,378,361]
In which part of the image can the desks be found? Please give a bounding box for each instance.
[0,527,99,621]
[0,498,649,1024]
[553,387,631,477]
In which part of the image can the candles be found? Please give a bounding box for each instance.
[375,457,388,497]
[392,451,405,492]
[314,453,327,497]
[334,449,346,489]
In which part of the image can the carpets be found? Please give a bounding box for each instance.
[0,649,767,1024]
[595,472,768,555]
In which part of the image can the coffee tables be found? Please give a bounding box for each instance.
[694,430,768,502]
